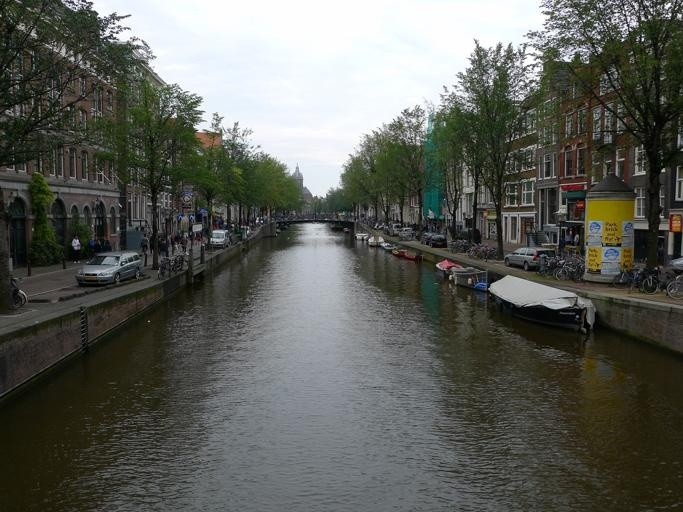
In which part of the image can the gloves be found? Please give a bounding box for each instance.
[436,259,597,335]
[343,228,421,261]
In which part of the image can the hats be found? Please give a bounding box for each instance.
[206,216,264,250]
[504,247,557,271]
[374,220,447,248]
[74,250,143,286]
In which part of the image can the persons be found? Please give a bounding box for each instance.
[85,239,112,262]
[71,234,83,264]
[564,230,572,246]
[349,214,441,241]
[574,230,580,246]
[141,214,269,255]
